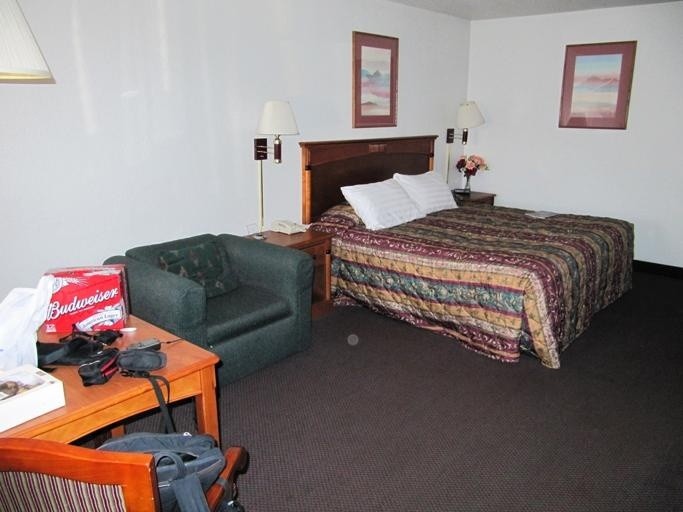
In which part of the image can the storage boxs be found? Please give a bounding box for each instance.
[40,263,131,335]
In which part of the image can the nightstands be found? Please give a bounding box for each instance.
[242,227,338,321]
[447,190,497,207]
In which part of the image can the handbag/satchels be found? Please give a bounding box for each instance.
[117,350,170,374]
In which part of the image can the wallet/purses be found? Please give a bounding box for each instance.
[76,347,120,387]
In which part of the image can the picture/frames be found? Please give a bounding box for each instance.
[350,29,400,130]
[556,39,639,130]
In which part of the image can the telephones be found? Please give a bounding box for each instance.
[272,219,306,234]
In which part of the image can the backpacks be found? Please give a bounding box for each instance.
[94,430,227,512]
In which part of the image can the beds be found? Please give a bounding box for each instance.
[296,133,637,370]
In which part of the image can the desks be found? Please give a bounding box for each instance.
[1,306,222,460]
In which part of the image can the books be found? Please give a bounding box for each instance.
[525,210,557,219]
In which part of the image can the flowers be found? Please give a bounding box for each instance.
[454,154,490,177]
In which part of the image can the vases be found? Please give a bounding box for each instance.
[464,175,471,194]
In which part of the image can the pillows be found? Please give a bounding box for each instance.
[338,177,424,230]
[159,238,240,299]
[393,170,458,216]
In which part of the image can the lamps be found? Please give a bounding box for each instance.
[252,97,301,166]
[446,100,485,145]
[1,0,52,83]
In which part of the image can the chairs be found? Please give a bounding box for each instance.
[98,232,316,388]
[0,429,252,511]
